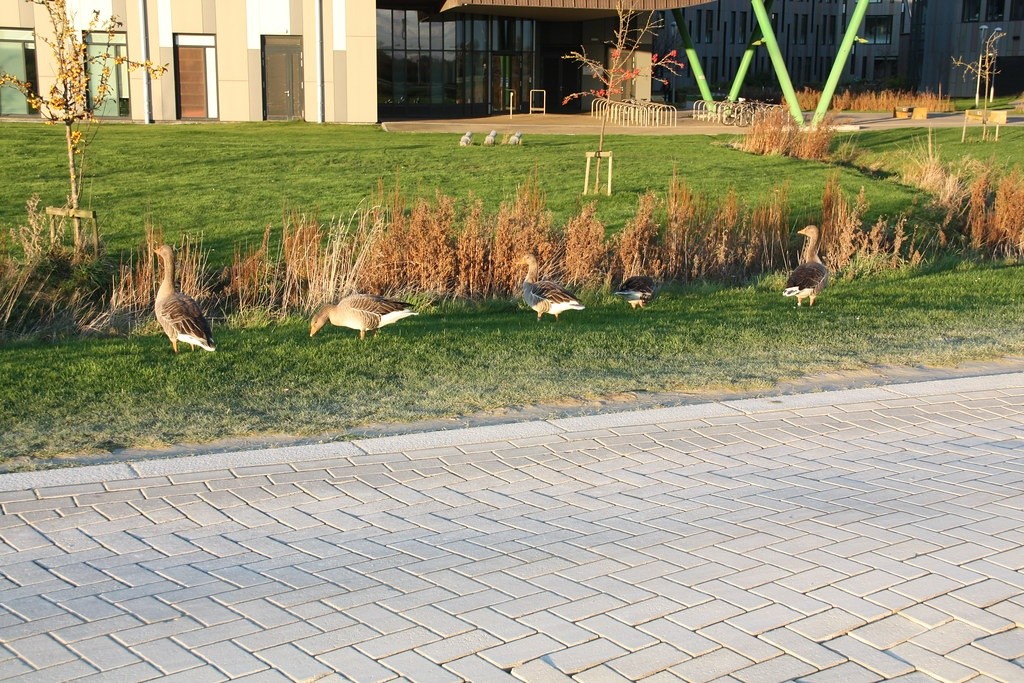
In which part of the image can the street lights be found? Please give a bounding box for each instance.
[975,26,989,109]
[989,28,1003,102]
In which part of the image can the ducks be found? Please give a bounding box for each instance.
[152,245,217,354]
[309,294,420,340]
[615,276,664,310]
[517,254,585,323]
[781,225,829,308]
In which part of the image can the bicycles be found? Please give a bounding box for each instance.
[720,100,751,127]
